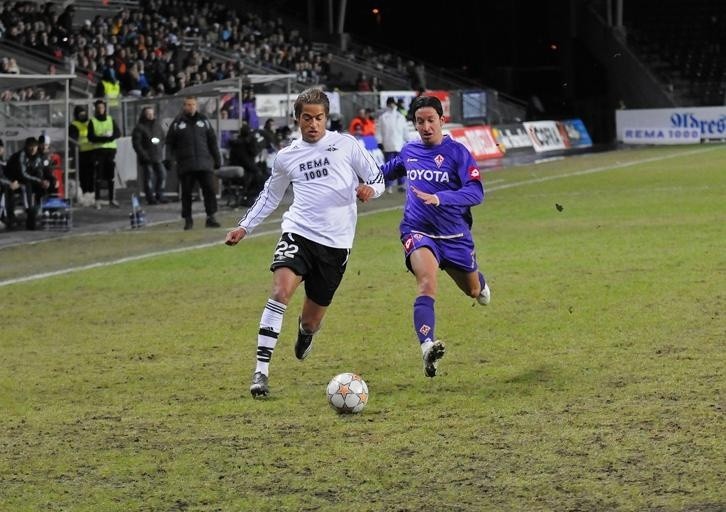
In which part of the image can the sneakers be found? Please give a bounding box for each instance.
[295,317,313,359]
[249,374,270,396]
[423,341,445,376]
[184,217,193,230]
[94,201,120,211]
[205,217,219,228]
[477,283,490,305]
[146,196,170,206]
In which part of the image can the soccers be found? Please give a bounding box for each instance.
[326,373,369,412]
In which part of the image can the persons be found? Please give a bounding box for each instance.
[223,89,386,397]
[2,1,427,230]
[377,96,490,378]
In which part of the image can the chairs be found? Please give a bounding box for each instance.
[223,153,252,208]
[40,193,72,233]
